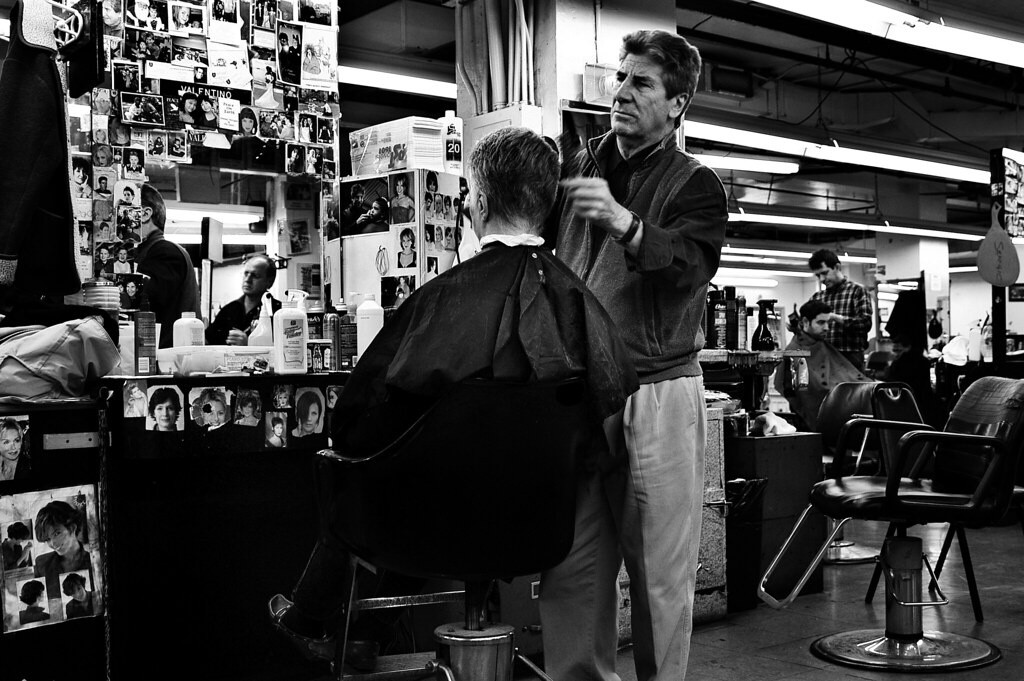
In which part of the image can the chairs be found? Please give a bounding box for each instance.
[757,377,1024,674]
[272,367,592,681]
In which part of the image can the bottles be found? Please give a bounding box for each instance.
[172,312,205,347]
[437,110,463,176]
[357,294,384,360]
[273,300,307,375]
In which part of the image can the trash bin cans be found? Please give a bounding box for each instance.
[725,476,770,617]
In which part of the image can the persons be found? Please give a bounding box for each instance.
[775,300,886,450]
[205,255,281,344]
[542,29,728,681]
[800,250,872,377]
[55,0,480,450]
[0,418,30,480]
[0,500,95,634]
[269,129,638,666]
[140,184,203,348]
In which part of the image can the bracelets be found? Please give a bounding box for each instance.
[611,211,640,246]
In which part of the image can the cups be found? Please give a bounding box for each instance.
[82,286,120,374]
